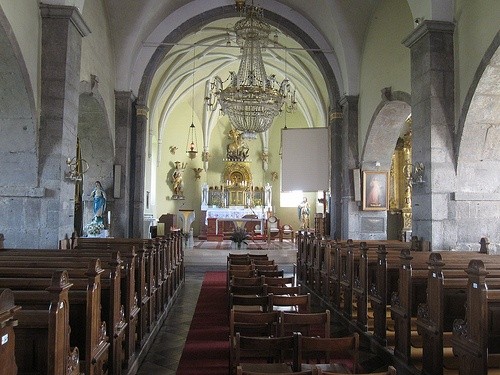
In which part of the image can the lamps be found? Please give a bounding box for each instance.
[402,162,427,185]
[278,48,289,159]
[188,43,197,160]
[205,0,297,140]
[64,155,89,182]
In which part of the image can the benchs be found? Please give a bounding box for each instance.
[301,232,500,366]
[0,221,185,375]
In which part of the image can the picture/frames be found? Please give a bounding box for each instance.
[361,170,390,212]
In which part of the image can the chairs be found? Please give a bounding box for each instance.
[226,252,360,375]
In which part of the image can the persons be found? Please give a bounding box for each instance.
[298,198,310,229]
[172,161,184,197]
[90,181,106,222]
